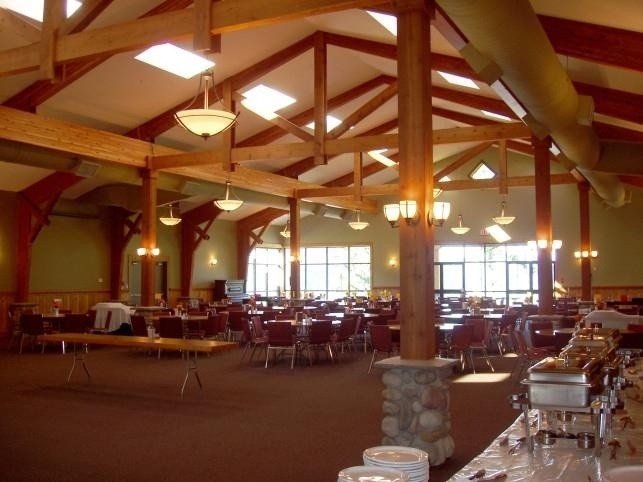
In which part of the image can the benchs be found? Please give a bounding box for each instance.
[37,330,239,400]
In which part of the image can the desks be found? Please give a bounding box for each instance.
[445,348,643,481]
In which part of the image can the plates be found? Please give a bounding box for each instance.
[337,446,430,482]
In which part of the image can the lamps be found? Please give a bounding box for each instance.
[136,247,161,257]
[348,209,370,231]
[212,177,243,213]
[383,201,451,229]
[172,55,240,140]
[159,202,182,226]
[280,218,290,239]
[451,215,472,235]
[491,203,516,227]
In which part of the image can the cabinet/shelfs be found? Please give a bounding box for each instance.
[214,280,246,303]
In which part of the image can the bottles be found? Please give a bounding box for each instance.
[148,321,155,341]
